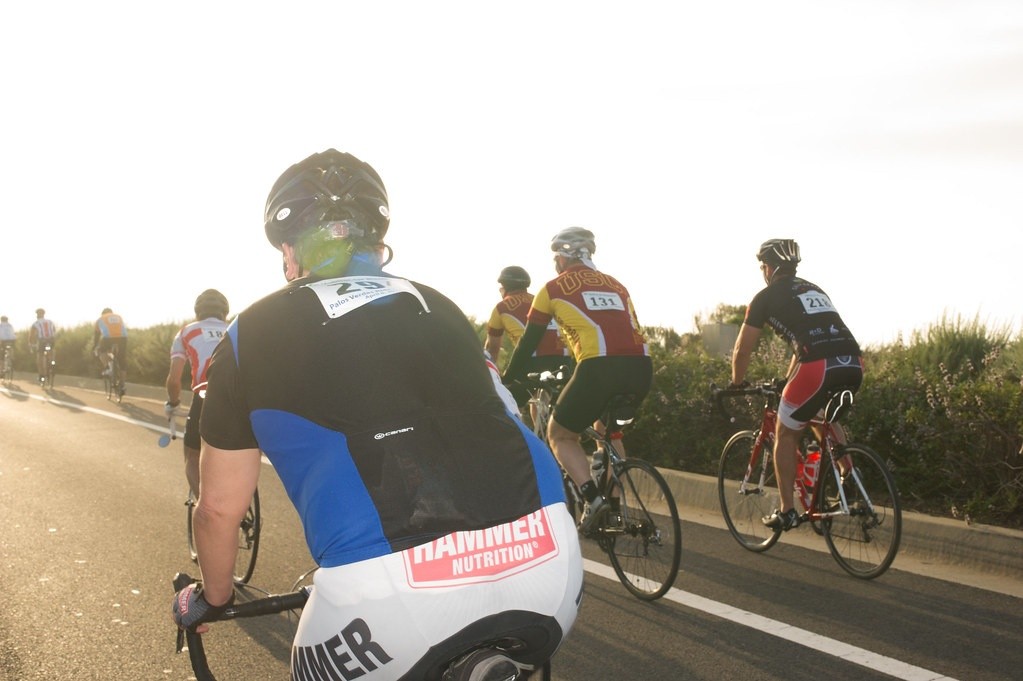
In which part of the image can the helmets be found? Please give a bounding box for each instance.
[36,309,44,317]
[1,316,8,322]
[102,308,113,314]
[550,227,595,254]
[195,289,230,321]
[264,147,390,253]
[757,239,801,262]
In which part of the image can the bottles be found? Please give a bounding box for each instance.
[803,440,819,494]
[589,447,604,487]
[795,451,805,493]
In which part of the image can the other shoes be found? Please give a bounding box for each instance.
[100,366,110,375]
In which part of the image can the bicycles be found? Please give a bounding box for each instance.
[165,409,264,586]
[92,345,125,403]
[708,377,903,582]
[498,364,683,602]
[30,344,55,389]
[171,567,549,681]
[0,345,16,388]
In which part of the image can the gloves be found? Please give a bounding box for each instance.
[164,397,179,417]
[726,380,751,396]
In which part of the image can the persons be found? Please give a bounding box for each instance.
[730,239,869,527]
[485,266,569,429]
[503,227,653,532]
[165,289,230,501]
[0,316,17,376]
[93,307,128,394]
[173,149,583,681]
[29,309,56,381]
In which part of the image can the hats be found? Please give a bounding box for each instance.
[496,266,529,287]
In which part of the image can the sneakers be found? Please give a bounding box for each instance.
[577,495,610,532]
[761,508,797,527]
[840,467,860,497]
